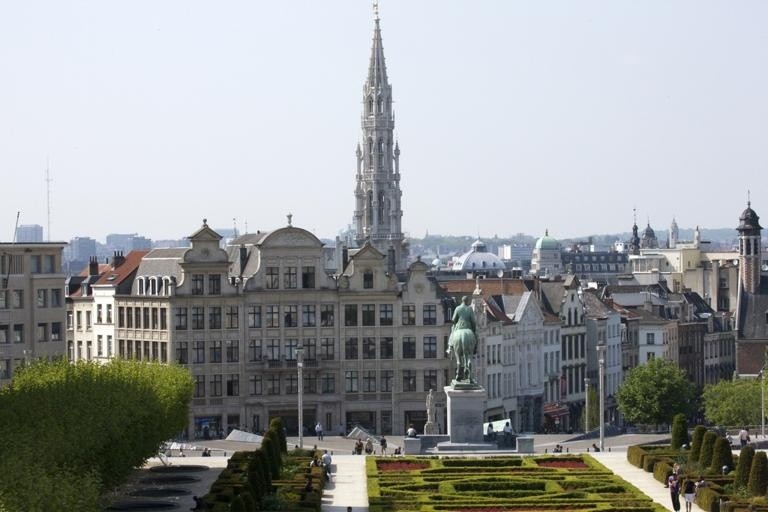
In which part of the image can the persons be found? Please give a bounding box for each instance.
[314,421,324,441]
[394,448,399,454]
[379,435,386,454]
[206,448,212,457]
[592,443,600,452]
[501,421,512,447]
[446,293,480,357]
[177,447,184,456]
[489,421,493,439]
[552,444,560,452]
[424,388,437,422]
[737,426,750,447]
[363,438,374,454]
[398,446,402,454]
[345,505,353,511]
[189,494,205,510]
[354,437,364,454]
[725,431,733,444]
[405,423,418,437]
[720,463,729,474]
[437,423,442,434]
[557,446,563,452]
[201,446,208,456]
[198,496,207,508]
[308,449,332,482]
[488,423,492,442]
[663,462,706,512]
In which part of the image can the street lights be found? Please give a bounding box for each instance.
[595,338,609,453]
[583,375,592,434]
[758,366,767,439]
[293,342,306,448]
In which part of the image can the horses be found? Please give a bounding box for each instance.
[452,328,476,384]
